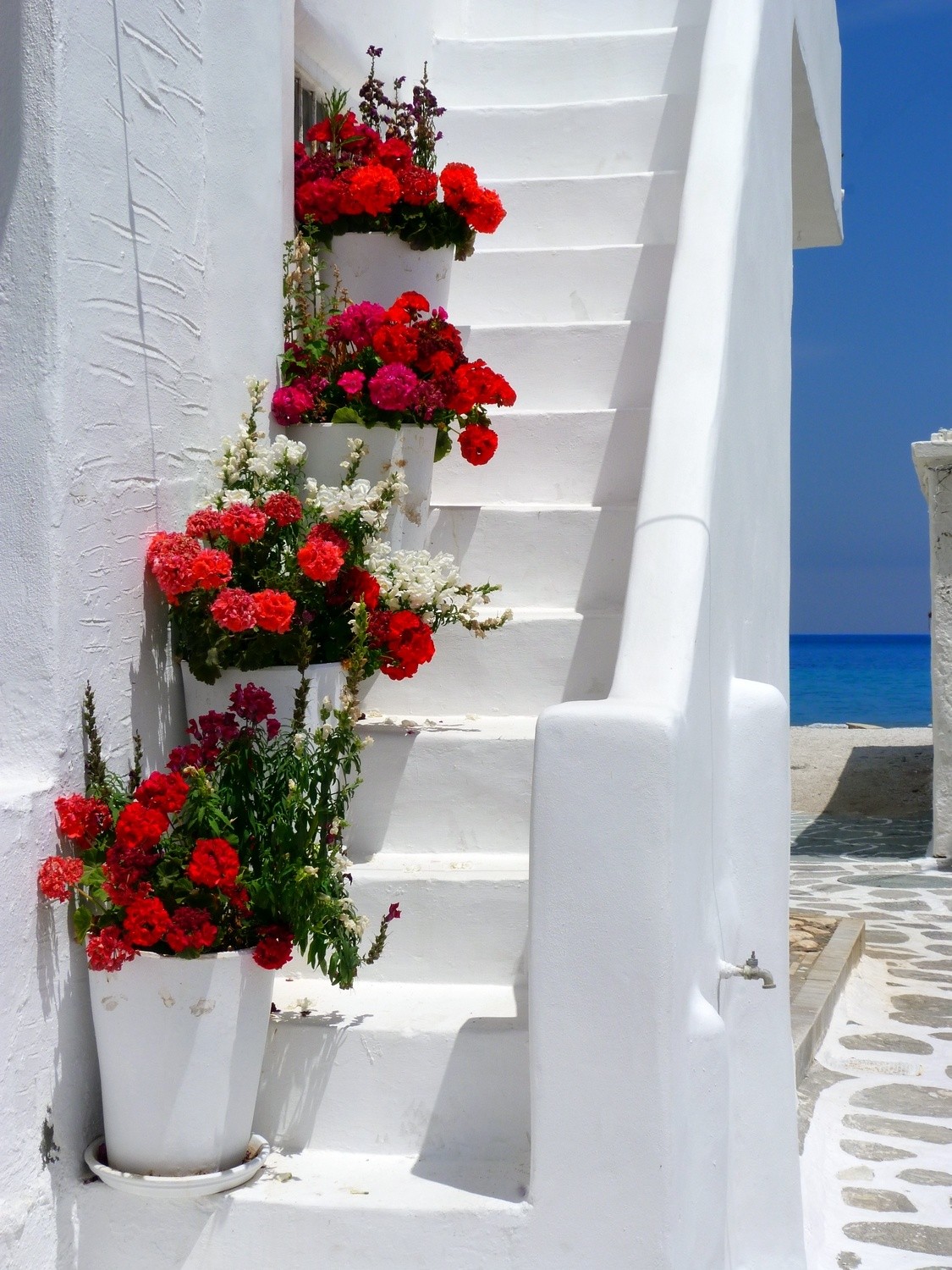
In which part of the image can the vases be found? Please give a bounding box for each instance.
[287,423,438,555]
[84,933,275,1178]
[316,231,456,353]
[180,659,349,845]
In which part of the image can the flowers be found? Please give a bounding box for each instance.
[146,377,515,685]
[40,590,400,989]
[272,213,515,465]
[294,44,507,262]
